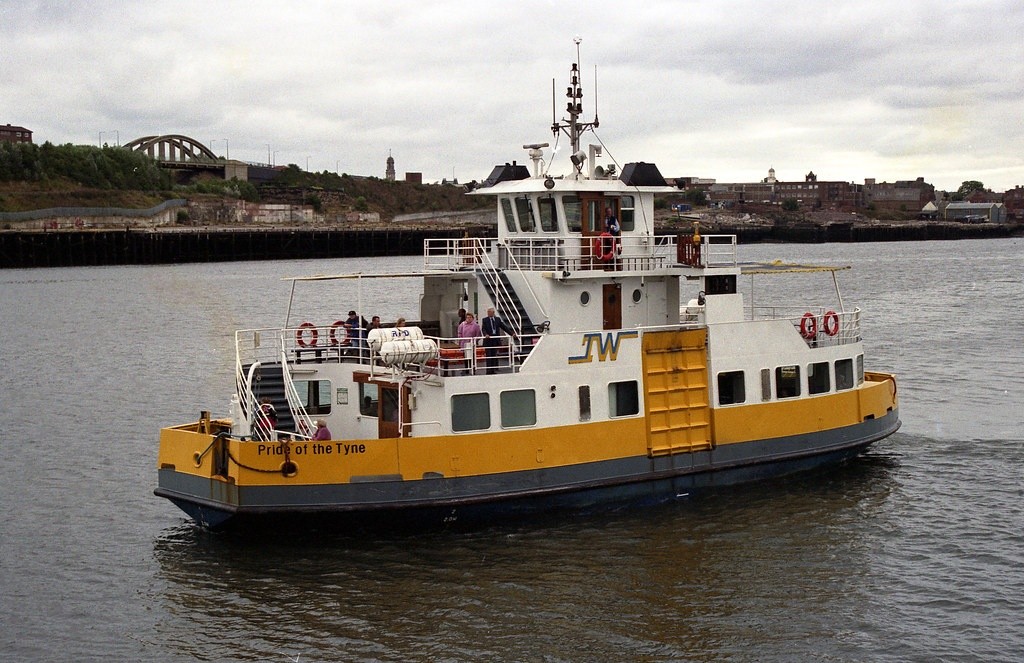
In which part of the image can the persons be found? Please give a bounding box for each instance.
[344,310,369,364]
[394,317,405,327]
[481,307,516,375]
[259,396,279,435]
[456,311,482,375]
[310,420,331,441]
[366,316,384,366]
[603,206,620,270]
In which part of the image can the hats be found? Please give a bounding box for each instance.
[348,311,356,315]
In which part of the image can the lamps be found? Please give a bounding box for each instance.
[570,150,587,174]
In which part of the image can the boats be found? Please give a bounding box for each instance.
[152,35,903,534]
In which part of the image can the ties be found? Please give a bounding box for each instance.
[491,318,498,330]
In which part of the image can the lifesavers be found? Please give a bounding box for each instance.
[800,311,817,340]
[330,321,351,345]
[594,231,619,262]
[823,310,841,337]
[679,235,700,265]
[296,322,319,348]
